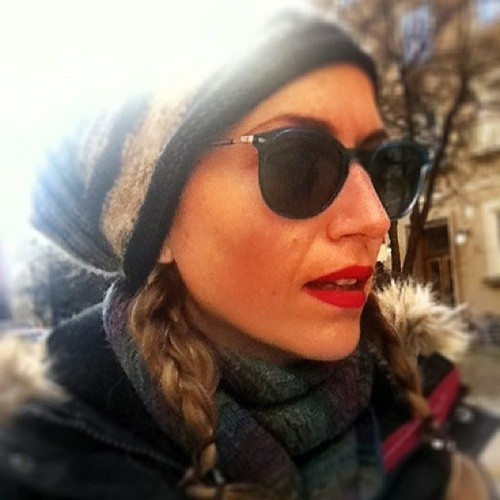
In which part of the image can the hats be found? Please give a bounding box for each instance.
[29,0,378,287]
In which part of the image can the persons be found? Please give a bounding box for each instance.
[1,13,498,500]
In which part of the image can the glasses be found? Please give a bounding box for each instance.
[206,127,433,219]
